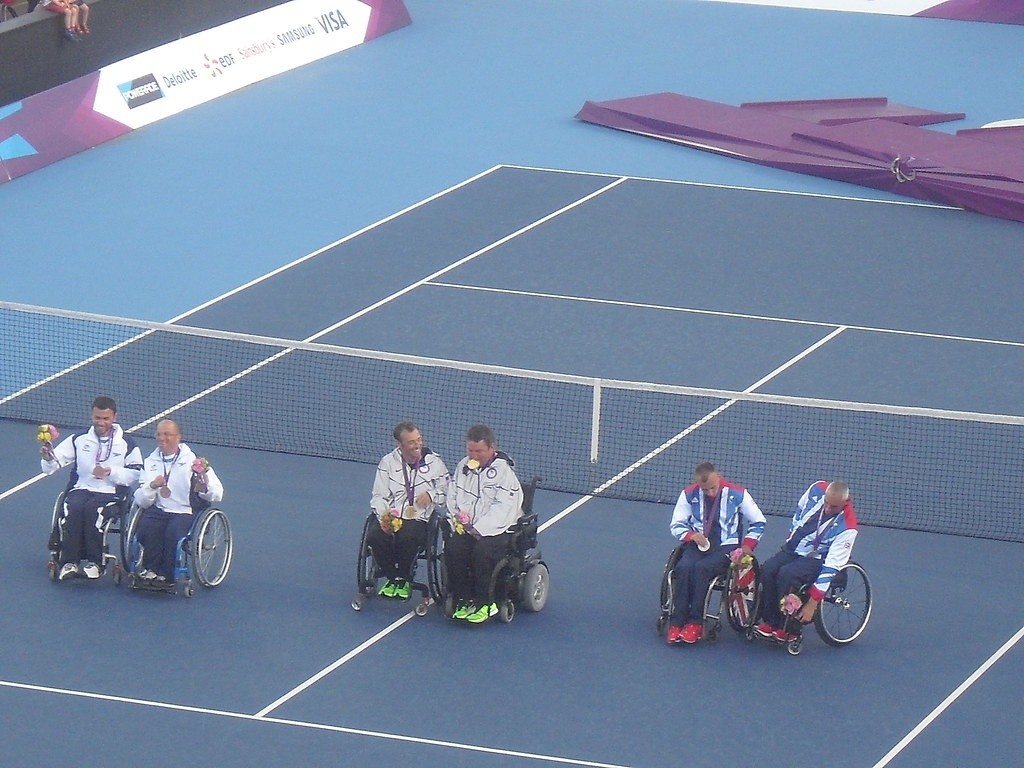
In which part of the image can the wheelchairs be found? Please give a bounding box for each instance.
[742,557,874,655]
[351,513,443,616]
[48,487,133,586]
[125,506,234,598]
[656,542,760,643]
[444,476,550,623]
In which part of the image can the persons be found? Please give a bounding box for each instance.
[366,421,451,600]
[39,396,143,581]
[42,0,90,39]
[446,425,524,624]
[752,480,858,642]
[667,462,767,645]
[133,420,223,589]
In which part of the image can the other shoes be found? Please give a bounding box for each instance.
[76,25,84,37]
[65,26,76,38]
[82,25,89,35]
[156,576,166,581]
[140,569,157,580]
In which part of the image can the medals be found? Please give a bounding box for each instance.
[698,538,710,551]
[467,459,480,470]
[807,551,817,559]
[405,506,417,518]
[160,487,171,499]
[93,466,104,476]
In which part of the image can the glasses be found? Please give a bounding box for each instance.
[153,431,179,438]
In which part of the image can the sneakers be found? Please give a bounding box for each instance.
[681,625,703,643]
[84,562,100,578]
[466,603,498,623]
[60,563,78,579]
[755,624,772,636]
[393,581,412,598]
[667,626,682,643]
[453,602,476,618]
[770,630,804,642]
[378,581,396,597]
